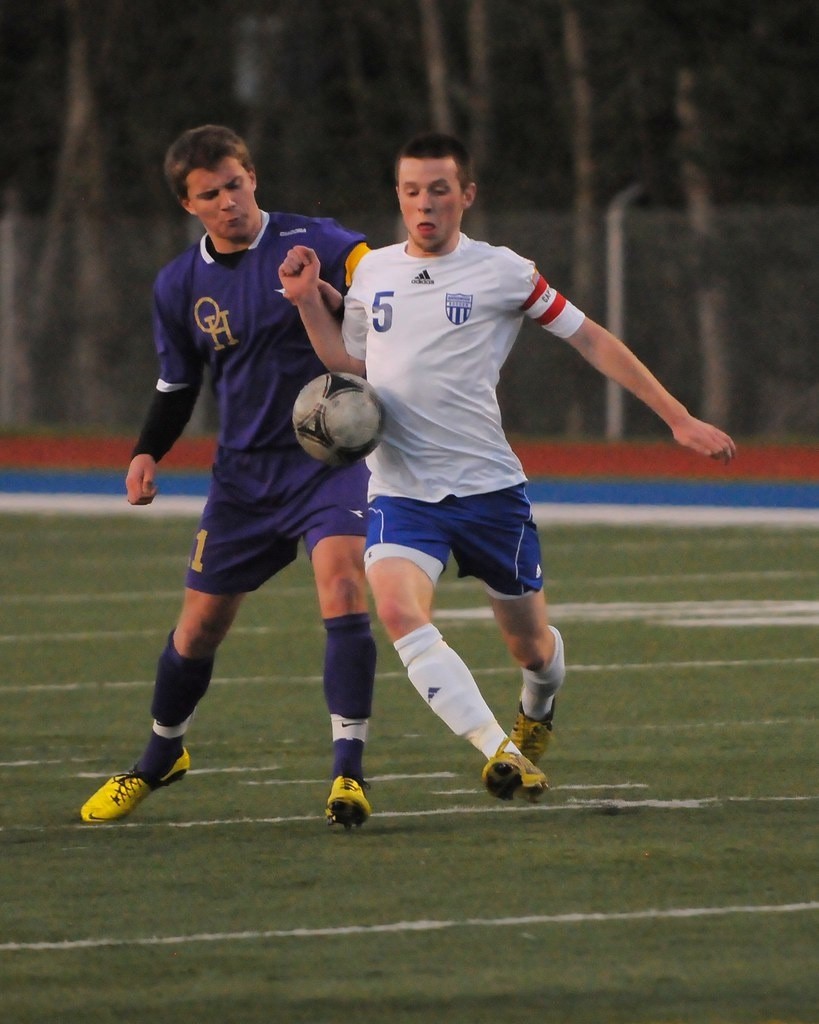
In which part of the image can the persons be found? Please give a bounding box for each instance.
[278,128,742,803]
[77,123,376,832]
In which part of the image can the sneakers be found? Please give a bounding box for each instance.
[80,747,190,822]
[481,738,549,802]
[325,774,372,830]
[508,684,555,766]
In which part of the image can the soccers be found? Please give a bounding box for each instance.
[293,371,386,465]
